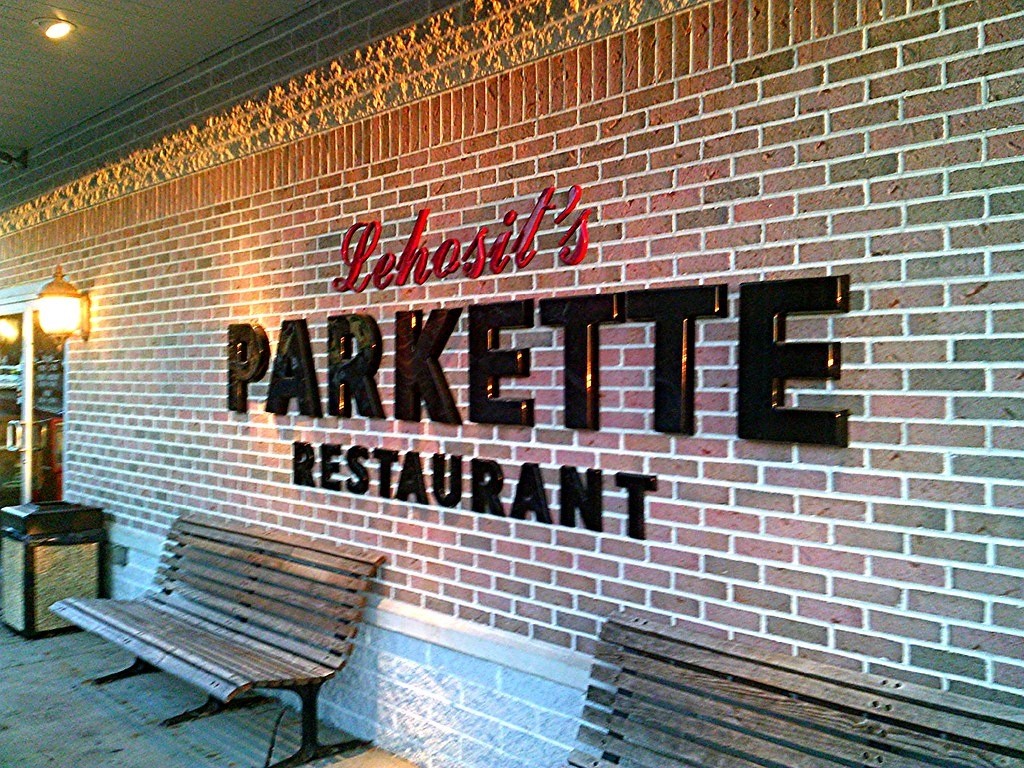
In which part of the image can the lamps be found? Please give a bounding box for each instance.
[40,13,72,41]
[36,265,90,352]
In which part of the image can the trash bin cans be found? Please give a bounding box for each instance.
[0,499,108,639]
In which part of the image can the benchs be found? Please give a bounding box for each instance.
[45,509,389,768]
[565,612,1024,768]
